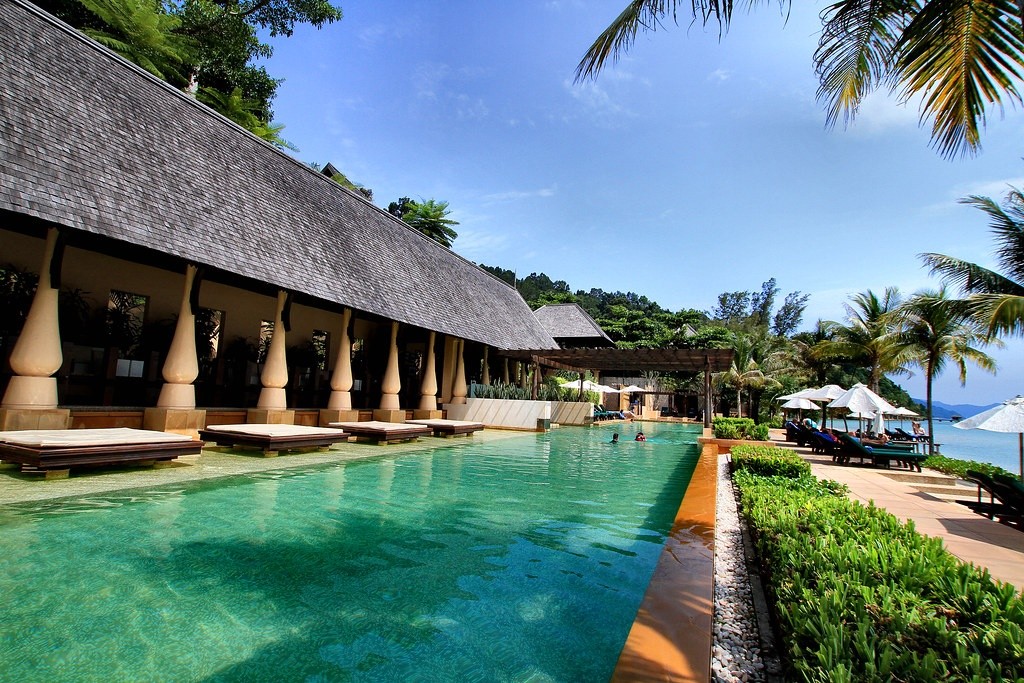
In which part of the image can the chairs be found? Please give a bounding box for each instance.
[784,421,929,472]
[967,470,1012,510]
[992,473,1024,515]
[594,405,621,422]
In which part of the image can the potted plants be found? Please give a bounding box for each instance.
[0,263,592,402]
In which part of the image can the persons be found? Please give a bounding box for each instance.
[607,433,620,444]
[630,419,634,422]
[619,410,626,419]
[635,432,645,441]
[786,418,926,446]
